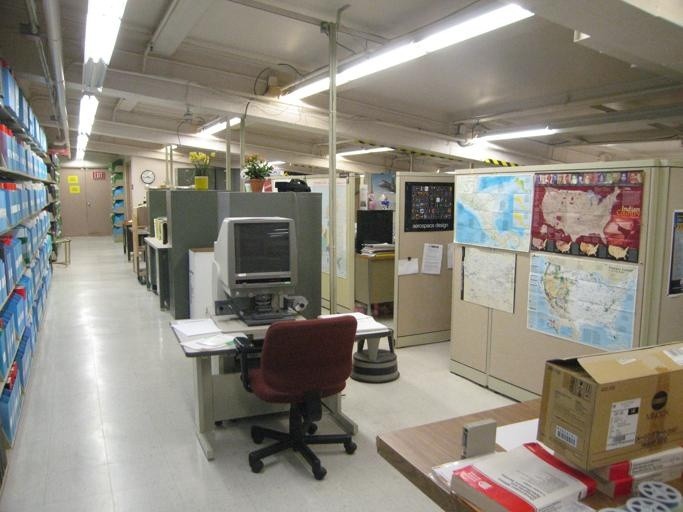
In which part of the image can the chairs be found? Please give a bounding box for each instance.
[232,315,358,481]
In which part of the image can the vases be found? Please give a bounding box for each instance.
[194,177,209,190]
[250,177,266,192]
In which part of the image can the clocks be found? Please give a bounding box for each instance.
[141,170,156,184]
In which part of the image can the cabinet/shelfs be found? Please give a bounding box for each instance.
[110,157,127,247]
[0,62,65,506]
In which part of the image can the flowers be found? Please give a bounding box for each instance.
[242,154,274,179]
[187,150,217,176]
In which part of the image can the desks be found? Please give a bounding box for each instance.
[167,311,393,462]
[373,398,683,511]
[354,251,394,317]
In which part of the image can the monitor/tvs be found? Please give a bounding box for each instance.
[213,215,299,292]
[356,210,393,252]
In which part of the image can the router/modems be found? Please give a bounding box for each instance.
[460,418,497,458]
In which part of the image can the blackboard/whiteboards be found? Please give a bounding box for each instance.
[189,247,216,320]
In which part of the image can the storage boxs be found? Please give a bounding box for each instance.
[534,339,682,474]
